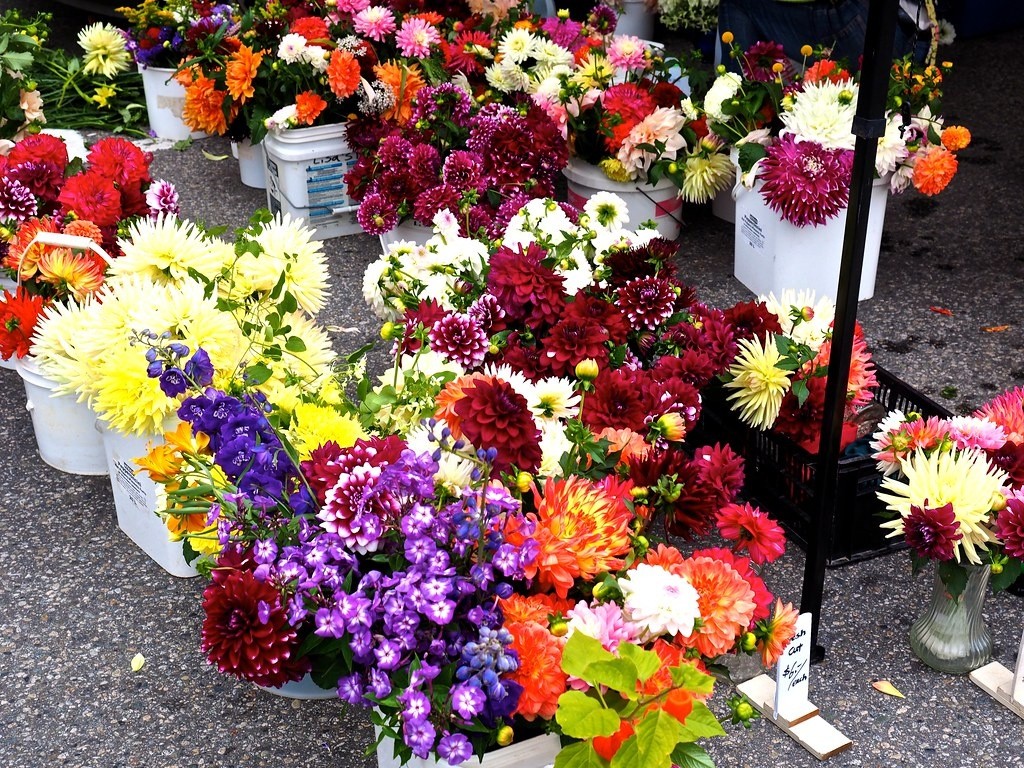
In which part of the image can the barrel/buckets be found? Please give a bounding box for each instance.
[139,1,889,313]
[0,270,564,768]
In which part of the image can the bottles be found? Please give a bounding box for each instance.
[907,555,998,675]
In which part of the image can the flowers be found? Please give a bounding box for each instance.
[1,0,1021,767]
